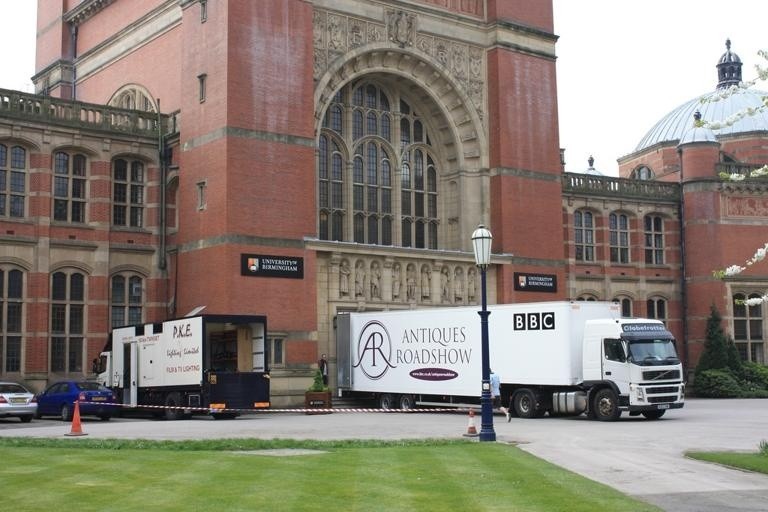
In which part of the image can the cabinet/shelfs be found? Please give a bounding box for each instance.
[211,328,253,371]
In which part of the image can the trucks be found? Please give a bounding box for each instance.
[334,295,688,425]
[78,304,271,423]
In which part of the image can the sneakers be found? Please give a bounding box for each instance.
[507,413,512,422]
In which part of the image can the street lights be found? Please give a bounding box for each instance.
[470,224,502,444]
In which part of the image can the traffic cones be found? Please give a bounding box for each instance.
[462,405,480,439]
[64,396,89,438]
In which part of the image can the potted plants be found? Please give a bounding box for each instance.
[304,368,333,414]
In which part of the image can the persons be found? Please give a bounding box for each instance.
[489,367,511,422]
[320,353,328,384]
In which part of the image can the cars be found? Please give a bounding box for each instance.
[0,380,39,423]
[33,380,119,424]
